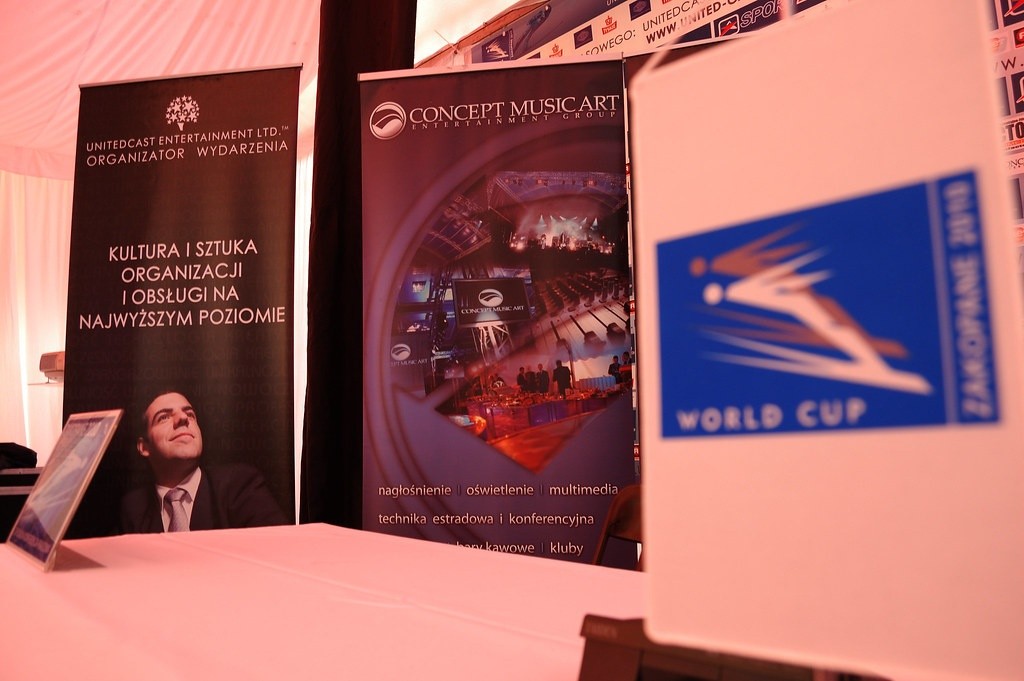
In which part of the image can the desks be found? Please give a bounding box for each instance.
[0,507,646,681]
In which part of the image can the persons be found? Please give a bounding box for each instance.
[113,383,288,538]
[464,351,633,402]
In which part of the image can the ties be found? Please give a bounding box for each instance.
[164,488,190,532]
